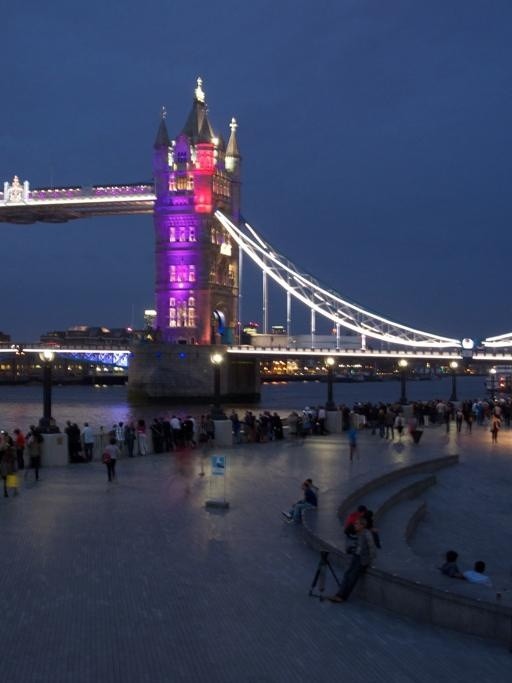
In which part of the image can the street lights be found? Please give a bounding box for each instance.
[488,368,496,400]
[208,350,227,419]
[396,359,410,405]
[33,350,61,434]
[324,356,337,411]
[448,361,458,400]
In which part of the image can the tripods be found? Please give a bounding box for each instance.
[309,562,341,602]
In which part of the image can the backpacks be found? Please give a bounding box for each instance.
[103,451,111,463]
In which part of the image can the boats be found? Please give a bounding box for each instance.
[337,373,366,382]
[393,368,420,381]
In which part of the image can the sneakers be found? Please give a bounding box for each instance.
[330,596,343,602]
[282,511,293,518]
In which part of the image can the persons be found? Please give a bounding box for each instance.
[345,511,382,549]
[340,397,511,460]
[230,404,328,444]
[462,560,494,587]
[344,505,367,527]
[282,477,319,518]
[327,517,376,603]
[1,413,218,500]
[441,550,465,580]
[287,482,317,525]
